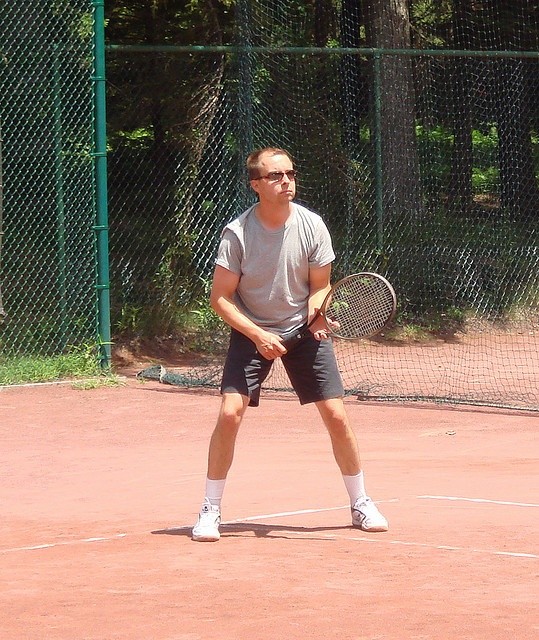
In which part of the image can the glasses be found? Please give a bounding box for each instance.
[251,170,296,181]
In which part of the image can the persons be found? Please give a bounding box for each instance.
[192,147,389,541]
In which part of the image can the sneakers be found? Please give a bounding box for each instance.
[192,498,221,541]
[350,495,388,532]
